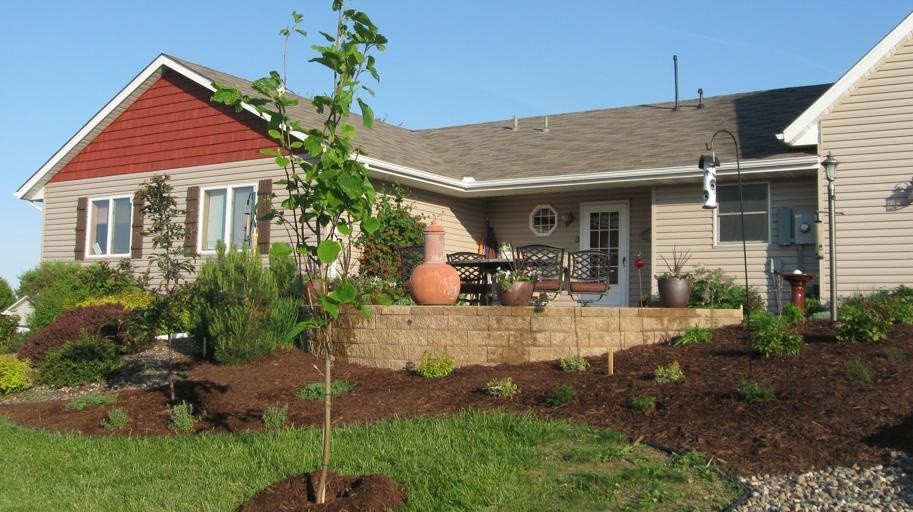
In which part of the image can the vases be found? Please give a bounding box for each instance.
[658,278,692,309]
[371,290,392,305]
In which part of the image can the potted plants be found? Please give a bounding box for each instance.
[303,273,329,304]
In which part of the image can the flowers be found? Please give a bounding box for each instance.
[653,245,697,288]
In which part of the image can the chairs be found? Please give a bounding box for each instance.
[398,244,612,306]
